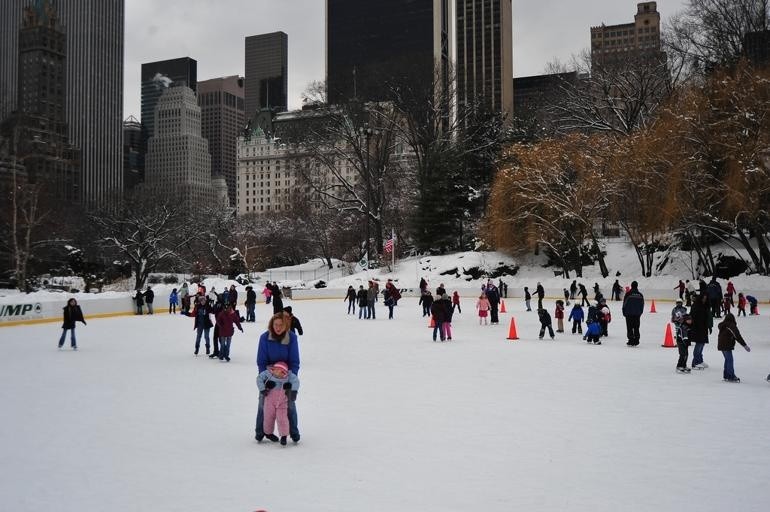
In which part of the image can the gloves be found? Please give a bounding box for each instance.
[283,382,298,400]
[260,379,276,397]
[744,345,750,352]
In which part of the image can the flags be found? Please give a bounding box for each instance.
[384,239,393,253]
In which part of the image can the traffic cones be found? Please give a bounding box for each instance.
[499,300,507,313]
[649,298,656,313]
[428,315,435,328]
[506,316,519,339]
[661,323,677,348]
[753,307,760,316]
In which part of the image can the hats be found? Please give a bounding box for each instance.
[273,361,289,378]
[683,314,692,320]
[435,294,448,300]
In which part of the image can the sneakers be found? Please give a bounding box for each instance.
[677,366,691,371]
[695,362,709,368]
[255,432,300,445]
[194,348,230,361]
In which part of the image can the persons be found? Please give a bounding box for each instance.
[524,286,532,311]
[537,308,555,340]
[673,276,757,319]
[381,279,401,320]
[254,312,302,442]
[143,286,154,314]
[419,277,461,342]
[476,278,508,325]
[169,278,289,361]
[717,312,751,381]
[675,312,692,373]
[58,297,84,349]
[611,279,623,301]
[555,280,611,345]
[257,363,300,445]
[690,292,713,369]
[131,289,145,314]
[284,304,305,337]
[532,282,545,310]
[622,281,644,346]
[344,277,379,320]
